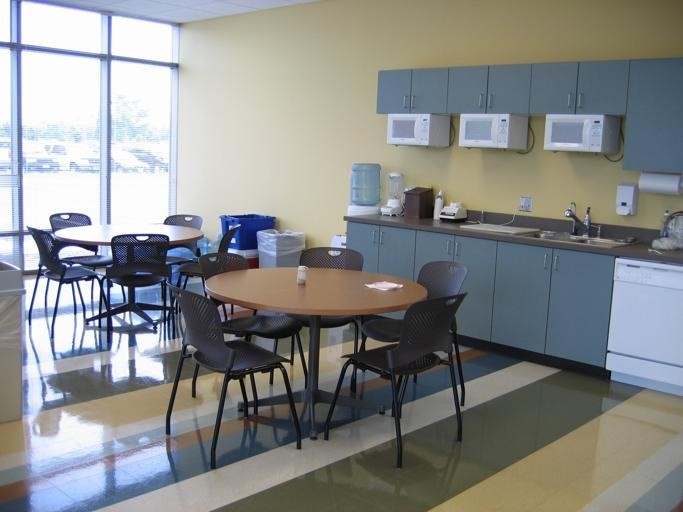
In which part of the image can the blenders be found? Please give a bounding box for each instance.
[380,173,403,217]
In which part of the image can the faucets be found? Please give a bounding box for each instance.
[563,205,591,236]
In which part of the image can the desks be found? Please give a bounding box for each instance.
[54,222,205,331]
[201,266,429,443]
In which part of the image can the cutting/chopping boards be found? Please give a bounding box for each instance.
[459,223,541,235]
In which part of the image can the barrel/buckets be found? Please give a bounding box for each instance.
[350,162,382,207]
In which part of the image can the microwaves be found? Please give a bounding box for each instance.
[385,114,451,149]
[457,113,529,153]
[542,113,620,156]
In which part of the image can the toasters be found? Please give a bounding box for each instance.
[438,205,468,223]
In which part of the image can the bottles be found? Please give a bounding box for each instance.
[661,210,670,238]
[583,210,591,237]
[295,265,307,285]
[433,189,443,220]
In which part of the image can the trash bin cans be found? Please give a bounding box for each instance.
[0,261,25,425]
[257,229,306,269]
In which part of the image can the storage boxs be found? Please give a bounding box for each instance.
[226,247,259,269]
[218,213,275,251]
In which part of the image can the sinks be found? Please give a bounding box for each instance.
[527,233,567,239]
[570,237,605,245]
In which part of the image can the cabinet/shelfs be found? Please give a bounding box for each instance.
[375,65,447,116]
[489,237,614,369]
[344,221,414,321]
[446,62,532,116]
[413,228,496,342]
[528,58,630,117]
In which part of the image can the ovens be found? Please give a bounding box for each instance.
[604,255,682,396]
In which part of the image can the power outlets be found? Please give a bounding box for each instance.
[518,196,533,212]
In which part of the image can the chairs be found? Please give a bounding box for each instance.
[23,225,110,340]
[159,282,303,470]
[267,246,364,394]
[321,292,468,469]
[189,251,307,407]
[44,212,114,315]
[346,261,467,407]
[104,231,176,342]
[172,224,241,290]
[163,214,203,232]
